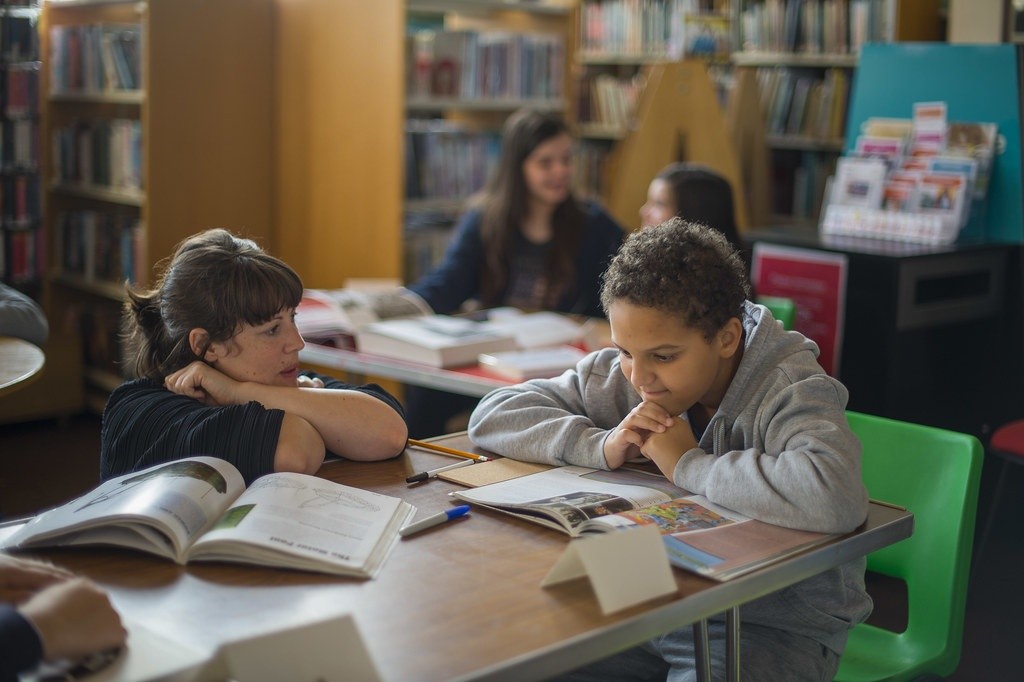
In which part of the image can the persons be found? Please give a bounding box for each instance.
[0,552,127,682]
[408,111,629,322]
[468,218,871,682]
[639,165,738,252]
[0,282,48,346]
[100,227,410,488]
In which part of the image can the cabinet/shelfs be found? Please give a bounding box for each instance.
[0,0,941,429]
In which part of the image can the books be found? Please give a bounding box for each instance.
[580,65,640,127]
[831,100,996,244]
[573,136,614,197]
[453,456,844,581]
[0,23,143,287]
[403,26,565,286]
[0,456,417,580]
[579,0,896,54]
[294,284,589,382]
[794,152,836,219]
[755,65,848,137]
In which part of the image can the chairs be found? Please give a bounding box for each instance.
[751,296,796,330]
[828,410,985,682]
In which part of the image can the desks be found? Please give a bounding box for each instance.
[283,324,593,397]
[0,430,915,682]
[0,334,47,399]
[734,224,1024,555]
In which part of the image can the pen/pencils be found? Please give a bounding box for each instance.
[399,505,472,538]
[407,438,493,461]
[406,458,476,483]
